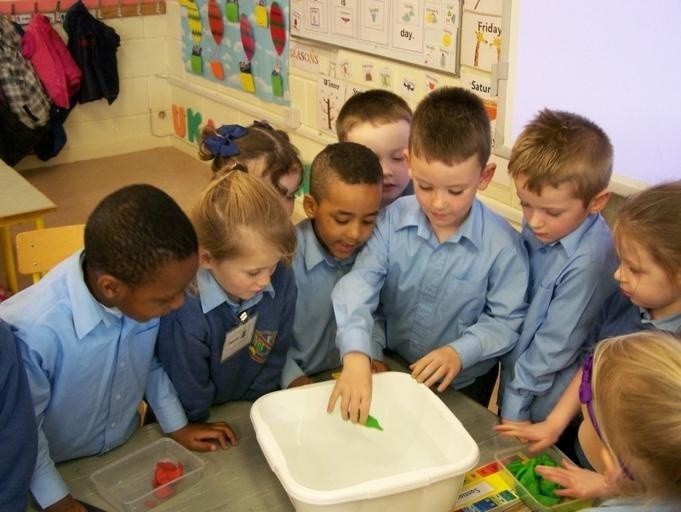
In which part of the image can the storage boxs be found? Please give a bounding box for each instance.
[249,372,481,512]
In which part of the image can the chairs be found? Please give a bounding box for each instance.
[13,224,87,284]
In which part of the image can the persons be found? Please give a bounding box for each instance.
[277,141,388,390]
[334,88,414,210]
[325,87,530,424]
[0,182,237,511]
[574,330,680,511]
[196,118,304,219]
[0,319,38,511]
[494,106,635,470]
[492,178,680,501]
[141,162,298,427]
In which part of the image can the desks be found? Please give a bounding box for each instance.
[0,160,59,286]
[28,355,602,512]
